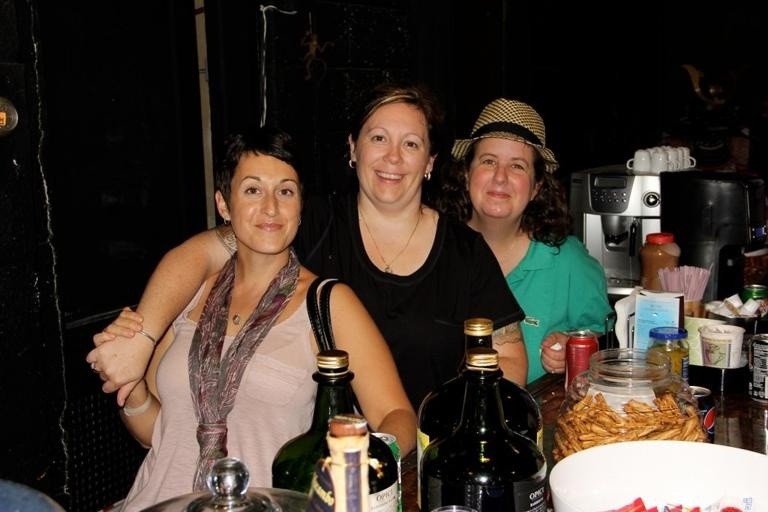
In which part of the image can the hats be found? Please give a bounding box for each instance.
[450,99,559,174]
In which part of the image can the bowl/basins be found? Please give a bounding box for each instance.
[704,310,767,352]
[547,439,766,511]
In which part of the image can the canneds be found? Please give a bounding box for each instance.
[690,385,716,443]
[370,432,404,512]
[564,331,599,392]
[745,334,768,404]
[646,327,689,386]
[742,284,768,304]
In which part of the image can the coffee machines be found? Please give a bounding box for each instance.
[570,172,742,316]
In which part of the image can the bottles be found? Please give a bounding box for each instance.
[273,350,402,512]
[546,348,713,472]
[419,317,544,454]
[323,410,373,512]
[418,350,548,511]
[717,245,742,299]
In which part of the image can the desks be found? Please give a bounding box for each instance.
[365,334,767,510]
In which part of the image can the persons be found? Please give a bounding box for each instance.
[449,96,614,385]
[83,85,531,445]
[94,125,422,511]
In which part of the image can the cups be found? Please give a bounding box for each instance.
[625,145,698,174]
[683,297,703,318]
[699,325,746,368]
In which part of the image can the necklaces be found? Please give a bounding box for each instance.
[231,258,287,324]
[357,203,423,277]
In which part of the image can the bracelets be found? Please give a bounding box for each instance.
[120,392,152,417]
[138,328,156,344]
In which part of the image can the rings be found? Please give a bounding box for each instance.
[551,368,555,375]
[89,362,99,371]
